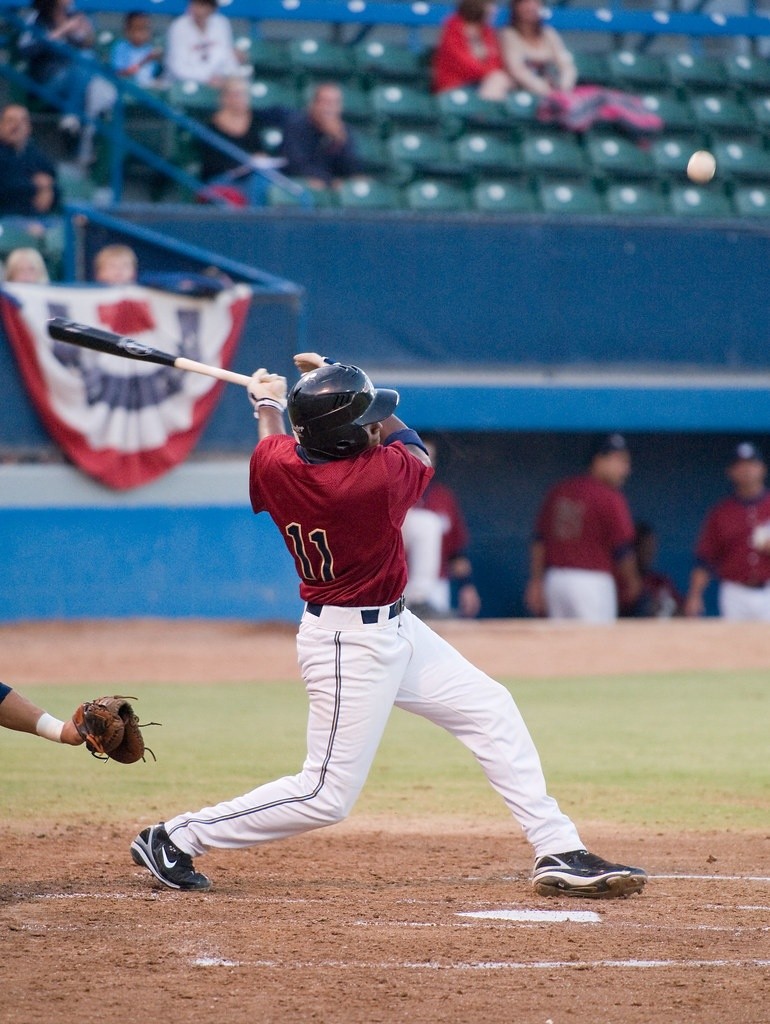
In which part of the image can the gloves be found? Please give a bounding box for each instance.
[293,353,342,374]
[248,368,288,415]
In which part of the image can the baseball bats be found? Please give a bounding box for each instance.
[46,316,269,389]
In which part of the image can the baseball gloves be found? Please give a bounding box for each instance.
[71,694,145,764]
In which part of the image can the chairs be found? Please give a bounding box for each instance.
[0,0,770,220]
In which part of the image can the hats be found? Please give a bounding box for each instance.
[593,432,629,458]
[723,441,764,466]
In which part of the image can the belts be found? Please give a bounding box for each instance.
[306,594,406,624]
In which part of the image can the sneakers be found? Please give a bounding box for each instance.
[531,849,648,897]
[130,821,212,891]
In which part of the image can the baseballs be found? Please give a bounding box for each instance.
[686,150,716,183]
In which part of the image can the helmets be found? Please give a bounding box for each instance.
[287,362,400,457]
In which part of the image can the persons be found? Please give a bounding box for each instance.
[0,104,65,216]
[18,0,106,154]
[110,10,164,88]
[162,0,245,86]
[127,355,647,898]
[3,247,50,284]
[91,244,141,284]
[0,681,162,765]
[430,0,513,102]
[626,524,686,616]
[519,430,644,621]
[499,0,580,100]
[681,442,770,619]
[284,82,368,211]
[188,74,293,205]
[399,440,482,620]
